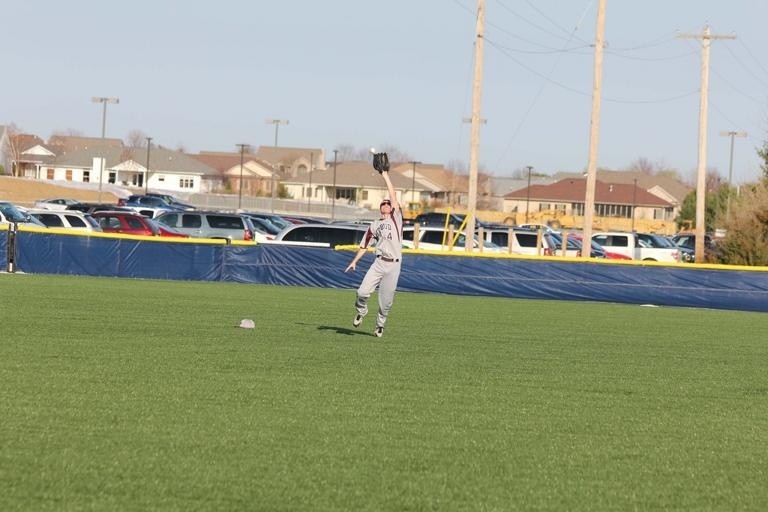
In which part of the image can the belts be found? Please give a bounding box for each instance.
[377,255,399,262]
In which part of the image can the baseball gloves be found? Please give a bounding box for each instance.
[373,152,389,173]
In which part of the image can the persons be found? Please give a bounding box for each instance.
[343,170,404,338]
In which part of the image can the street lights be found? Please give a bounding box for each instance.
[407,161,421,202]
[460,117,488,125]
[235,144,252,209]
[719,130,748,221]
[525,166,533,222]
[142,137,153,195]
[329,148,339,220]
[87,95,120,202]
[263,118,291,146]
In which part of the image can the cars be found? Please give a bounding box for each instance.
[0,193,714,264]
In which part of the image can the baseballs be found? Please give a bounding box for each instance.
[369,148,376,154]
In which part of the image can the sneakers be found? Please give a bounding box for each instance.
[353,309,368,327]
[374,327,384,338]
